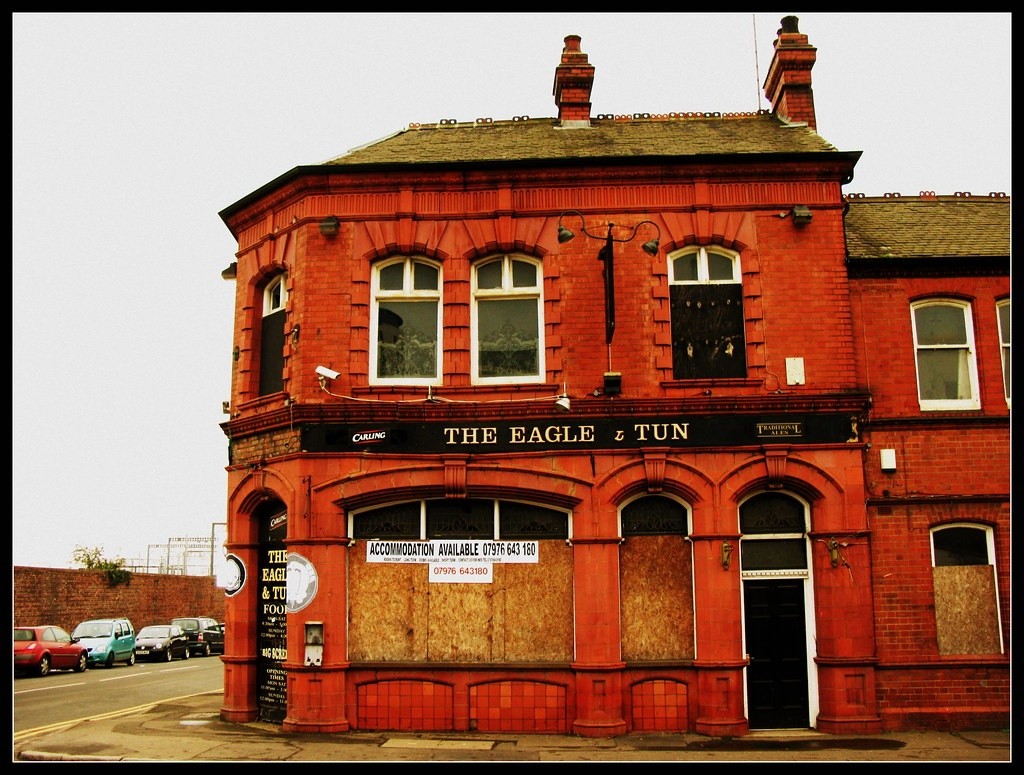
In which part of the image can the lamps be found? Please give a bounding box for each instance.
[793,206,813,224]
[554,382,570,413]
[222,262,237,279]
[320,216,340,234]
[558,211,661,256]
[423,384,438,403]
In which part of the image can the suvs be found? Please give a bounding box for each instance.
[171,616,218,631]
[71,617,136,668]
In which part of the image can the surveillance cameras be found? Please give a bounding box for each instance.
[315,366,341,380]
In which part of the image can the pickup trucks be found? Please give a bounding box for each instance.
[184,623,225,656]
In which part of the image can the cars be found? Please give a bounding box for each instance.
[14,626,89,677]
[133,625,190,662]
